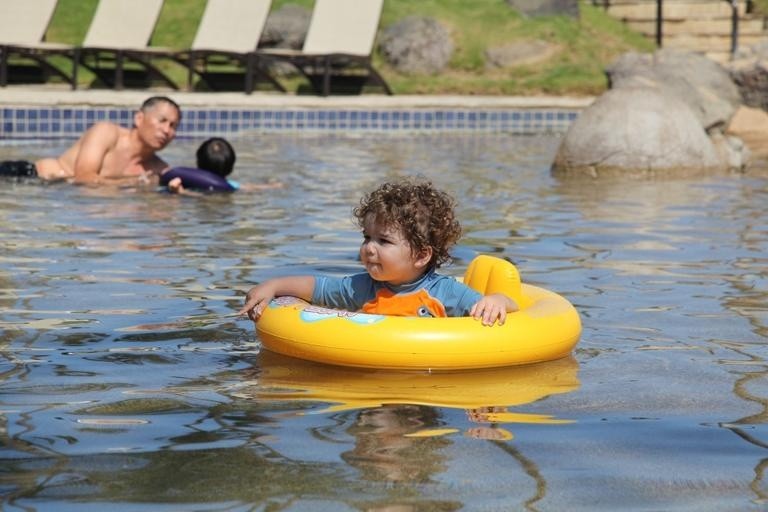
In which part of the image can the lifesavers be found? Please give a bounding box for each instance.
[255,255,581,370]
[161,167,235,193]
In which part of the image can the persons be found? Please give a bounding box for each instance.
[0,98,183,195]
[238,183,518,326]
[170,137,282,198]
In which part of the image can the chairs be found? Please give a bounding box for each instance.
[0,0,60,88]
[113,0,275,94]
[0,0,165,90]
[239,0,396,96]
